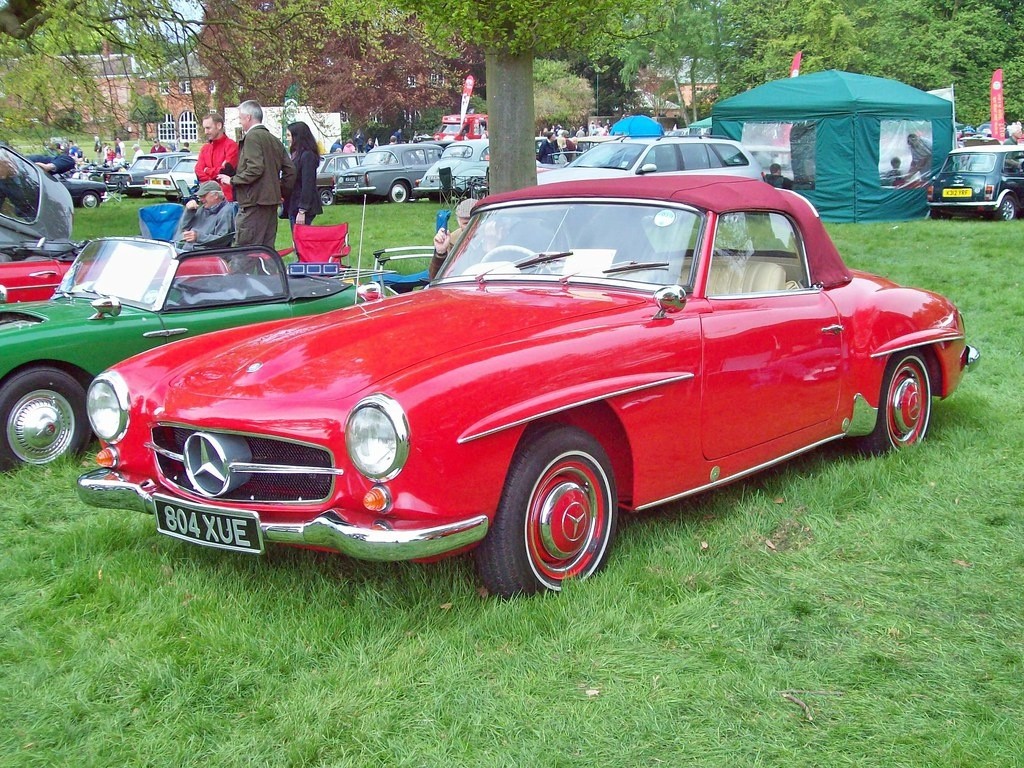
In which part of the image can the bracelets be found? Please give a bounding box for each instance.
[298,208,308,214]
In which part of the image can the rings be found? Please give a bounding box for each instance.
[296,222,298,224]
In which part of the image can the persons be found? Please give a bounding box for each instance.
[48,130,190,172]
[0,142,76,226]
[429,198,504,283]
[559,208,675,288]
[886,157,916,182]
[907,133,932,180]
[195,114,239,218]
[216,100,297,275]
[170,181,235,253]
[278,121,323,263]
[328,122,488,169]
[1002,121,1022,173]
[538,120,612,164]
[765,164,793,190]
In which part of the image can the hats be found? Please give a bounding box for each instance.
[55,143,60,149]
[154,138,159,142]
[193,180,222,196]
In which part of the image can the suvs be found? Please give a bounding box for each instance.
[537,135,768,186]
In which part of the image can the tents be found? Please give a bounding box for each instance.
[688,116,712,136]
[610,114,664,136]
[712,69,953,223]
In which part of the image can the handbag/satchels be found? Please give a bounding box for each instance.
[277,199,289,219]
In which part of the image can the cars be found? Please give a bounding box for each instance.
[73,173,982,604]
[1,234,383,475]
[0,113,619,306]
[926,144,1024,221]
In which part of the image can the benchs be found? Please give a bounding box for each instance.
[593,252,799,295]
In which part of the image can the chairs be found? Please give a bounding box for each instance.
[101,173,125,202]
[138,204,184,243]
[439,167,491,210]
[248,222,352,277]
[175,201,241,254]
[373,209,452,296]
